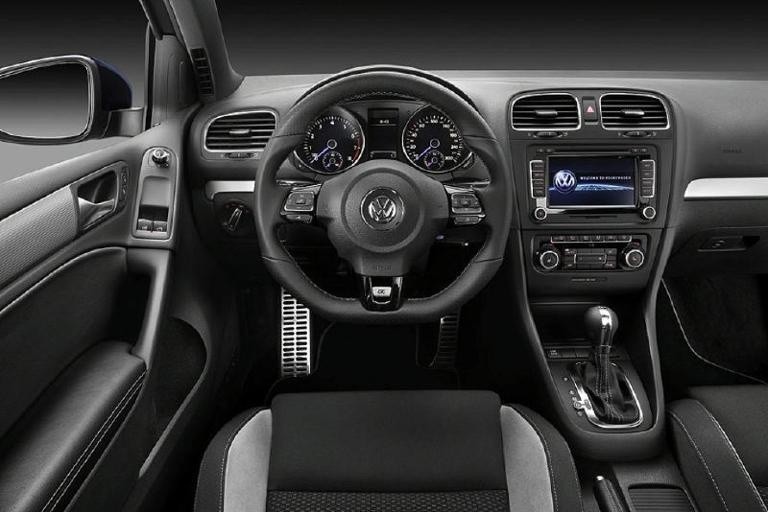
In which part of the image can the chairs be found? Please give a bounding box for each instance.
[666,384,768,509]
[195,389,582,511]
